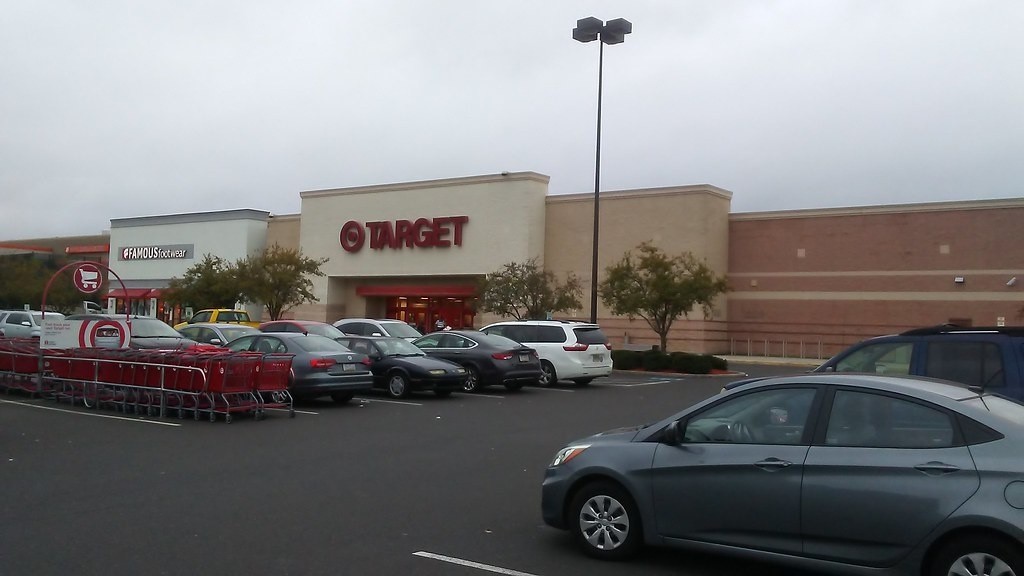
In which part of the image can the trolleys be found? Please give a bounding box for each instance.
[0,334,296,425]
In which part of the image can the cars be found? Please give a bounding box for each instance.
[410,329,541,395]
[334,335,469,400]
[176,323,272,352]
[259,318,366,350]
[542,370,1024,575]
[217,330,374,404]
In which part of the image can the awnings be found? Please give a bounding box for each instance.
[105,288,150,298]
[146,288,178,297]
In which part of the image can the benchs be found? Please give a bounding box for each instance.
[622,343,659,351]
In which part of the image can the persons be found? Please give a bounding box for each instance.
[409,316,417,330]
[435,318,445,331]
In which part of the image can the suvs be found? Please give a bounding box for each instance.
[0,309,67,339]
[323,317,439,348]
[459,316,614,387]
[715,322,1024,434]
[65,312,204,351]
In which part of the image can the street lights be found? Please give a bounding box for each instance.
[571,16,634,324]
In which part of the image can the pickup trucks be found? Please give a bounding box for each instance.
[173,307,266,335]
